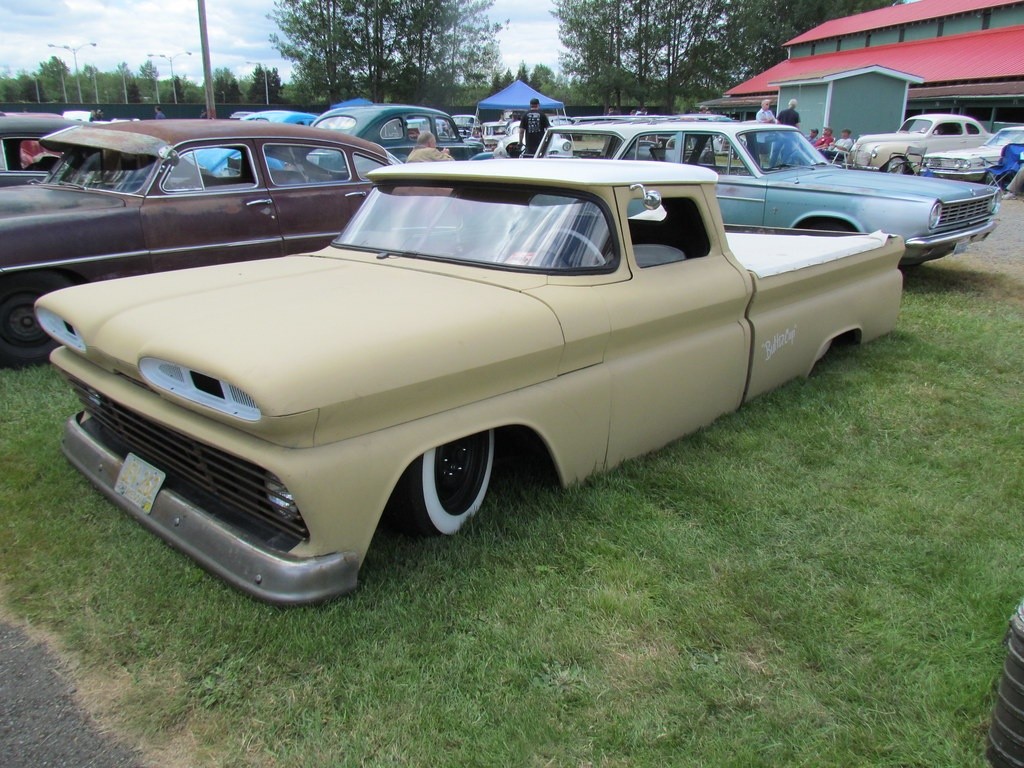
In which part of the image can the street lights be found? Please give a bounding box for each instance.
[147,51,192,104]
[247,62,269,105]
[48,41,97,104]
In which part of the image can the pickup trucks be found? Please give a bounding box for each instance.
[31,158,905,608]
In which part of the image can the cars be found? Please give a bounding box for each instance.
[0,112,102,188]
[0,119,455,369]
[920,126,1024,187]
[844,112,1007,174]
[179,102,573,182]
[531,122,1000,273]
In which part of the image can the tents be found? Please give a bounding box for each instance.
[329,98,375,111]
[471,80,576,150]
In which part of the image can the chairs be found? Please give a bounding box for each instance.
[987,143,1024,197]
[878,146,928,176]
[832,139,856,169]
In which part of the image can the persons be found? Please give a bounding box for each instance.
[607,105,710,116]
[405,133,456,161]
[498,112,520,126]
[804,127,853,163]
[1000,166,1024,200]
[464,118,475,139]
[154,105,168,120]
[199,110,208,119]
[770,98,802,169]
[517,98,554,158]
[88,109,105,122]
[756,98,776,171]
